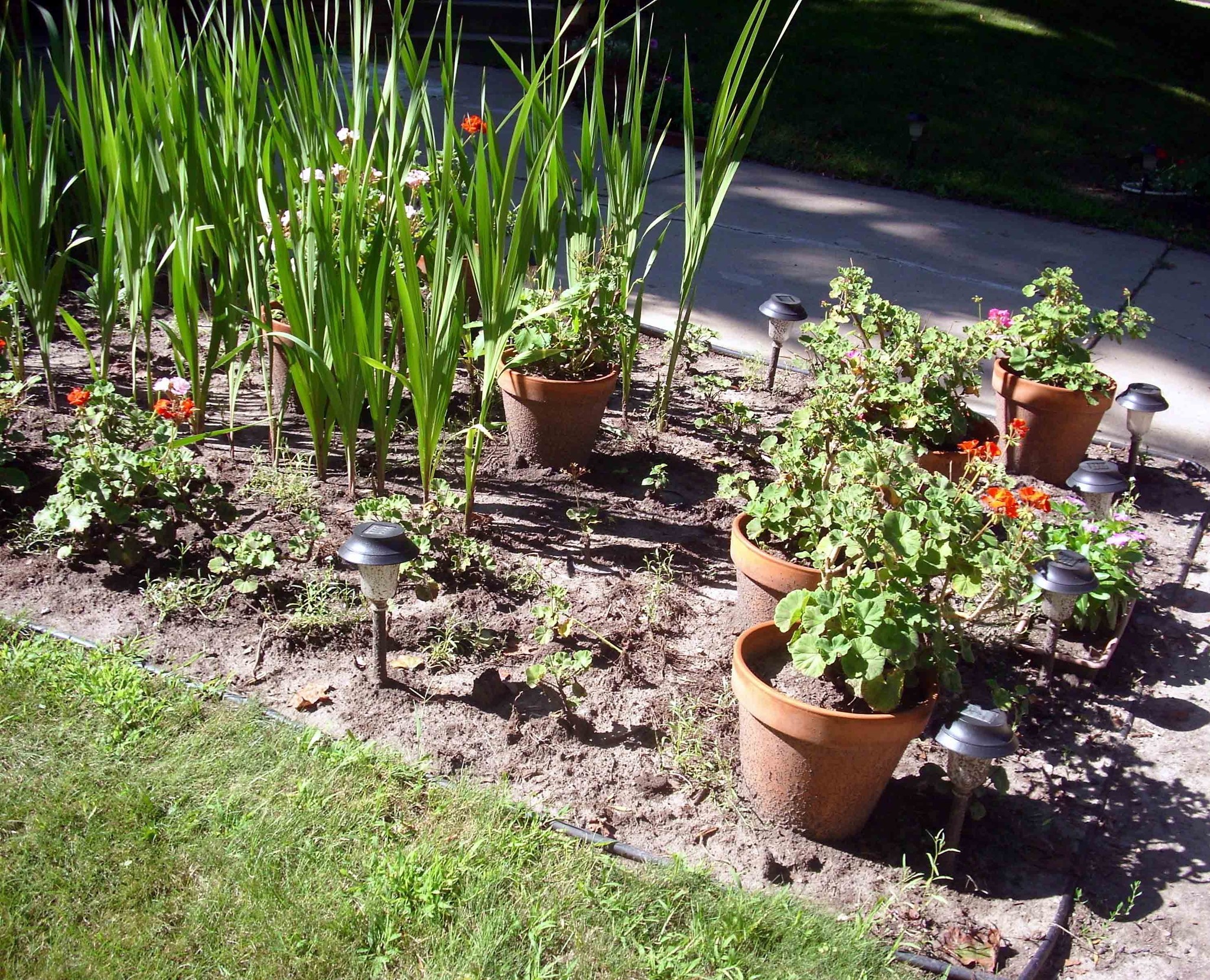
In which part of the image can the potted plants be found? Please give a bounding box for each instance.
[493,221,646,466]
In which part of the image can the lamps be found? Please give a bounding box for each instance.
[1112,381,1170,437]
[1027,548,1101,623]
[758,285,808,343]
[1065,456,1128,518]
[337,519,420,610]
[924,696,1022,798]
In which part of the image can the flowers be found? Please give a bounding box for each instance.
[727,255,1157,702]
[262,115,492,329]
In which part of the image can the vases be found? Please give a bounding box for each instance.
[992,356,1119,494]
[732,619,942,842]
[726,510,853,632]
[256,300,308,415]
[882,407,1010,487]
